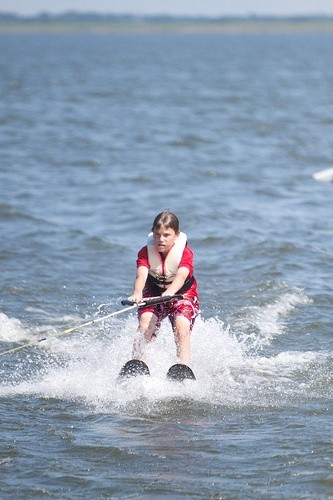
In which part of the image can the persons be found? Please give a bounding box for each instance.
[125,208,200,368]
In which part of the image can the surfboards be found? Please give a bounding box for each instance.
[118,360,197,379]
